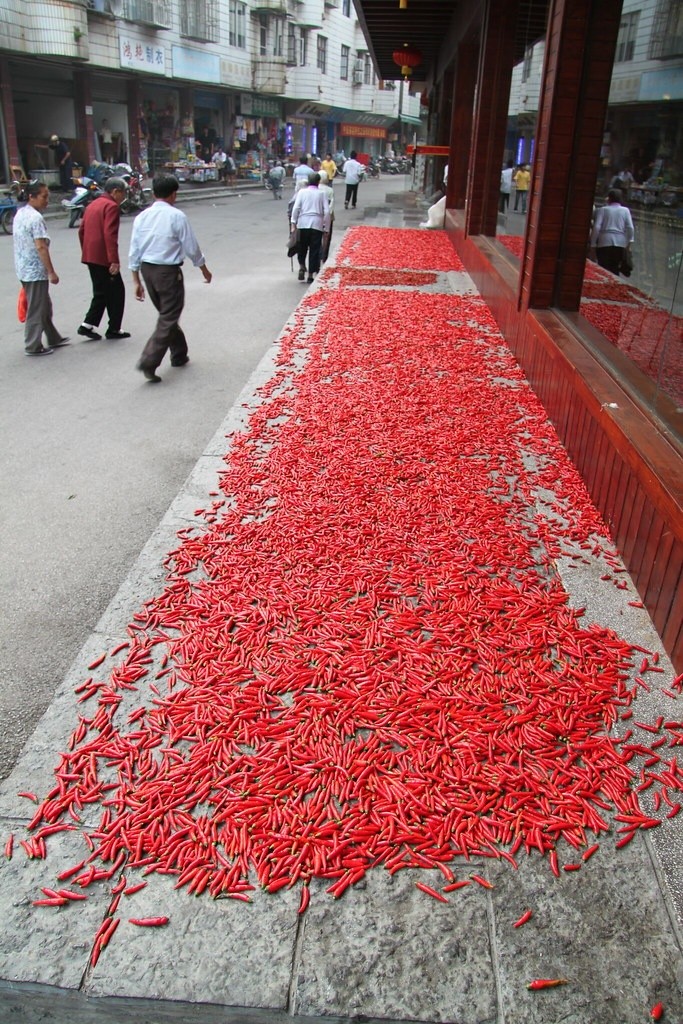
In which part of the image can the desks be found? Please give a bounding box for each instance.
[161,164,217,183]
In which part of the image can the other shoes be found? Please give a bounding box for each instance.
[77,325,101,340]
[25,348,53,356]
[514,209,518,213]
[105,328,130,339]
[307,278,313,282]
[523,210,527,215]
[49,336,70,348]
[171,355,188,367]
[298,269,304,280]
[137,360,161,383]
[352,205,356,208]
[345,200,348,209]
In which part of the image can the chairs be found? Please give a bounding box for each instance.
[9,164,33,192]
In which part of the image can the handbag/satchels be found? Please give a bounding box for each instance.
[621,248,633,277]
[287,224,298,257]
[18,284,53,322]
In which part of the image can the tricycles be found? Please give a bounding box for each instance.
[0,178,40,236]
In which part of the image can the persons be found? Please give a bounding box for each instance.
[498,159,635,278]
[199,128,236,181]
[128,174,211,383]
[100,119,113,165]
[76,176,130,338]
[35,134,73,191]
[265,151,364,284]
[11,182,69,355]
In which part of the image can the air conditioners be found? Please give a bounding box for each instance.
[354,59,364,83]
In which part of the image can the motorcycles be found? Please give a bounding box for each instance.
[61,175,106,227]
[368,155,413,179]
[91,161,153,215]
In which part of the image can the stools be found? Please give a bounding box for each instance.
[225,174,237,187]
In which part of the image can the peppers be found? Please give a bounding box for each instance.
[4,266,682,968]
[525,980,562,990]
[498,234,682,411]
[651,1001,663,1023]
[335,225,467,273]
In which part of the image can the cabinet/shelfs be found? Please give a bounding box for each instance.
[147,148,171,178]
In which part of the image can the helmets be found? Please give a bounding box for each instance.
[50,135,58,141]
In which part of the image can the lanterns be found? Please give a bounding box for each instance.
[393,43,421,74]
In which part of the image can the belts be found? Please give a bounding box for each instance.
[142,262,178,268]
